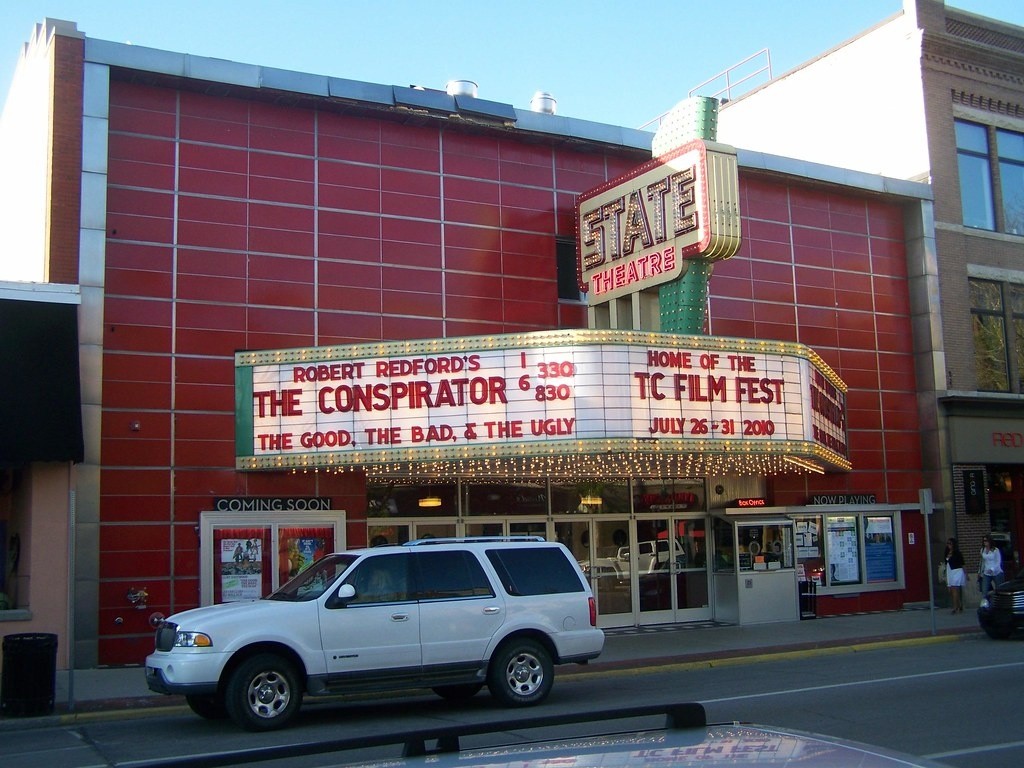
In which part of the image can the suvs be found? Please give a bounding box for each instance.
[144,536,609,732]
[577,539,688,585]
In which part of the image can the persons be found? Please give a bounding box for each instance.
[353,568,400,603]
[233,539,259,563]
[421,533,436,539]
[371,535,390,547]
[944,537,967,615]
[980,534,1005,593]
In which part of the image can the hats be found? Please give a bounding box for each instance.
[246,541,252,545]
[238,543,241,545]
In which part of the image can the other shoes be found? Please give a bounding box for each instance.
[951,608,958,615]
[959,604,963,613]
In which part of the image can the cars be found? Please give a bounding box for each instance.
[976,571,1024,638]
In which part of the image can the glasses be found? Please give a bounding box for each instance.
[982,539,988,542]
[946,542,953,545]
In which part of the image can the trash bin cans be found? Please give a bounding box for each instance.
[0,632,59,719]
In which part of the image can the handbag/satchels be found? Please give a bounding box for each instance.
[977,576,994,592]
[937,561,947,583]
